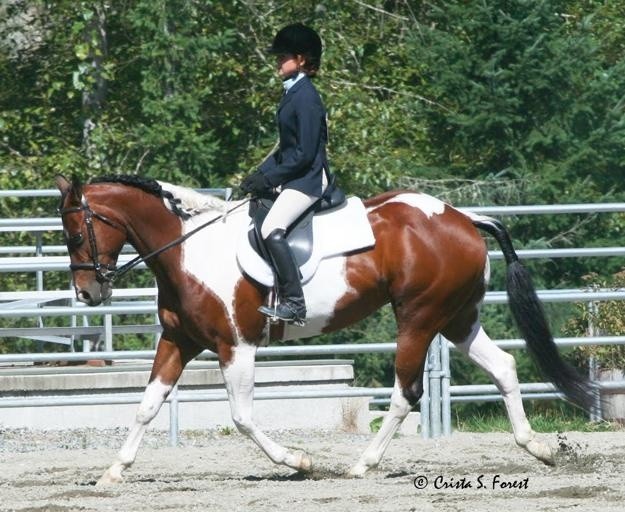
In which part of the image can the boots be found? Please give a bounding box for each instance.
[255,228,306,320]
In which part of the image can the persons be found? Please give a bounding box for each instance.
[240,23,331,327]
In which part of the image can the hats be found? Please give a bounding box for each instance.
[266,25,321,65]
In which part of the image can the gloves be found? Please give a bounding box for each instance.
[239,170,272,196]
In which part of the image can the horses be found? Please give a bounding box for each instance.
[49,168,617,487]
